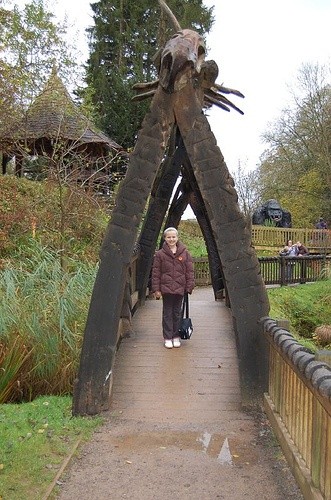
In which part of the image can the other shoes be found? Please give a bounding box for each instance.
[173,339,180,347]
[164,340,173,348]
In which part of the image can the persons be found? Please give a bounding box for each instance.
[293,241,308,284]
[149,227,196,349]
[278,239,298,286]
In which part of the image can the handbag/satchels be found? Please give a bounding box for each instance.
[180,318,193,339]
